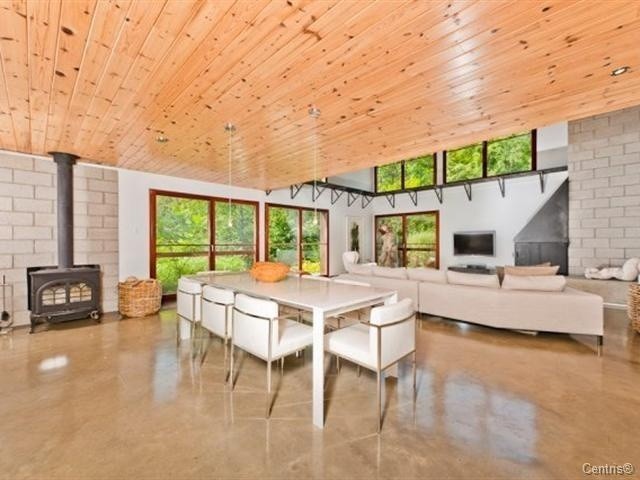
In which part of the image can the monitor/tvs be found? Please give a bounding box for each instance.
[452,230,497,260]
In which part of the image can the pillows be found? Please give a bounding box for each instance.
[348,262,567,292]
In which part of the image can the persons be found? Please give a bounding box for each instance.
[350,221,360,264]
[583,257,640,285]
[378,224,398,270]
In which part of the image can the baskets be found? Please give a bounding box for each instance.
[626,282,640,335]
[117,275,163,320]
[248,261,291,284]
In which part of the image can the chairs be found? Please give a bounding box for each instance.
[175,268,417,434]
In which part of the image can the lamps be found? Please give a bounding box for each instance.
[225,124,235,228]
[309,107,319,226]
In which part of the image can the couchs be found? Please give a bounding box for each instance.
[331,261,604,358]
